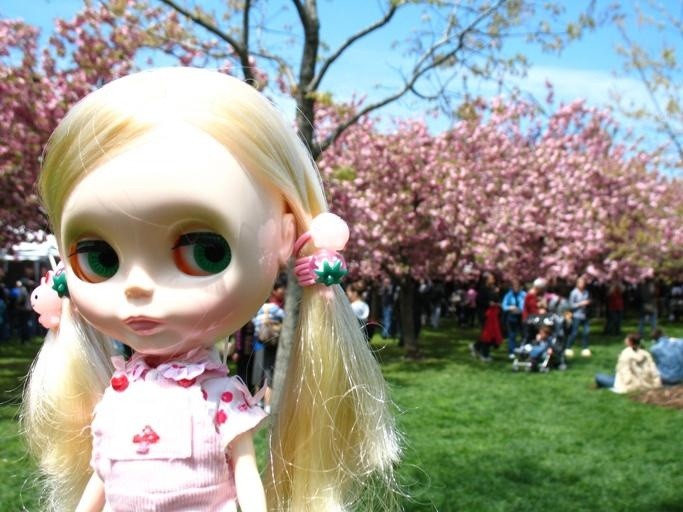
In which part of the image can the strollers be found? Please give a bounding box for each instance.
[511,312,568,372]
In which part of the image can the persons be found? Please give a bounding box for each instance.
[595,329,683,395]
[342,270,590,375]
[0,265,54,340]
[605,269,658,334]
[21,65,398,512]
[233,282,286,399]
